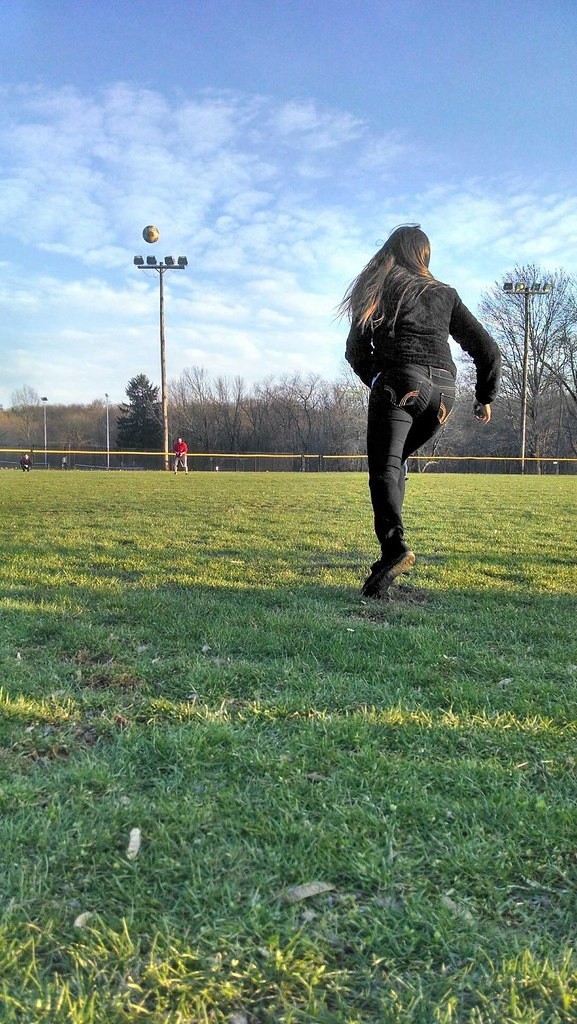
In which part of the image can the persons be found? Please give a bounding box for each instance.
[20,456,26,471]
[61,454,68,470]
[330,223,502,599]
[173,437,188,475]
[22,454,32,472]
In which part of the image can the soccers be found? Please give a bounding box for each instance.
[142,226,159,243]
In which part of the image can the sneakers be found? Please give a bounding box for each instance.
[361,530,415,599]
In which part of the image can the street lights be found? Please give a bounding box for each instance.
[133,255,189,469]
[41,396,48,468]
[104,392,109,469]
[503,282,553,474]
